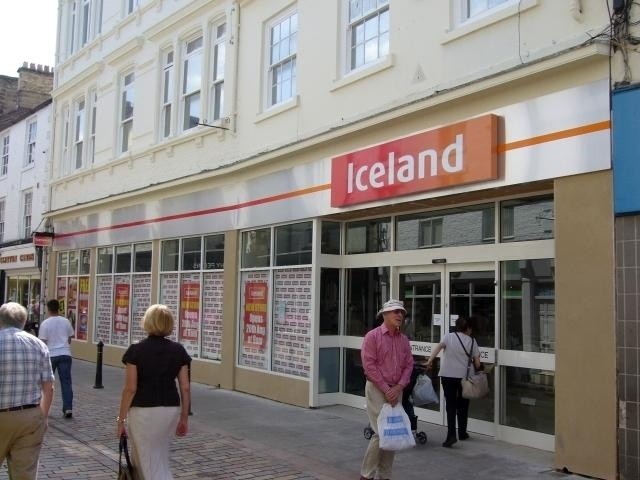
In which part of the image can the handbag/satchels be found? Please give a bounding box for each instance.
[412,374,441,407]
[116,463,137,480]
[376,399,419,452]
[461,370,490,401]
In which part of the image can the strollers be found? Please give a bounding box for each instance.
[362,360,429,445]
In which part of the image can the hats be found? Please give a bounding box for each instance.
[376,299,407,318]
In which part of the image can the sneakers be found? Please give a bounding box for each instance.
[64,410,73,418]
[458,432,470,441]
[443,436,458,447]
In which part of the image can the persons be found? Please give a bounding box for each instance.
[117,303,192,480]
[360,299,415,480]
[0,302,55,480]
[38,299,75,418]
[423,316,480,447]
[27,299,36,323]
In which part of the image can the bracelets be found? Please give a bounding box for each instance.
[117,416,125,423]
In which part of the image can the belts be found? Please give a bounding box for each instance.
[0,404,39,413]
